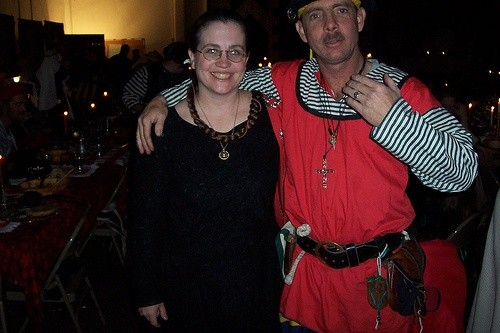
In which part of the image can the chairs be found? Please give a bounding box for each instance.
[19,163,127,333]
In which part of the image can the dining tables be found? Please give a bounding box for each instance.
[0,142,130,333]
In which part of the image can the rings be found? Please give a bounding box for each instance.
[354,92,360,100]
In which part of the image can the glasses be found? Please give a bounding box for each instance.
[195,48,248,63]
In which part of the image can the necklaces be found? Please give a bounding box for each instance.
[316,59,366,189]
[197,93,240,160]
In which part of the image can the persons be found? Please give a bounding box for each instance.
[124,9,289,333]
[0,81,26,175]
[29,46,67,148]
[428,86,487,245]
[136,0,479,333]
[108,45,141,82]
[122,42,191,114]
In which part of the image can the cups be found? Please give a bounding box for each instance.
[26,118,117,181]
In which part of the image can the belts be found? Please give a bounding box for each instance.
[296,216,417,270]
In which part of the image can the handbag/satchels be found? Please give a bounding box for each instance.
[387,241,426,316]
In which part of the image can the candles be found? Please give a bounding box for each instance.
[490,106,494,126]
[498,98,500,113]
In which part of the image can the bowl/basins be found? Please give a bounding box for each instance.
[20,178,59,197]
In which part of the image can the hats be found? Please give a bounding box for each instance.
[0,70,28,101]
[286,0,367,21]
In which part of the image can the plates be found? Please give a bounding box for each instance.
[12,205,60,223]
[468,121,500,149]
